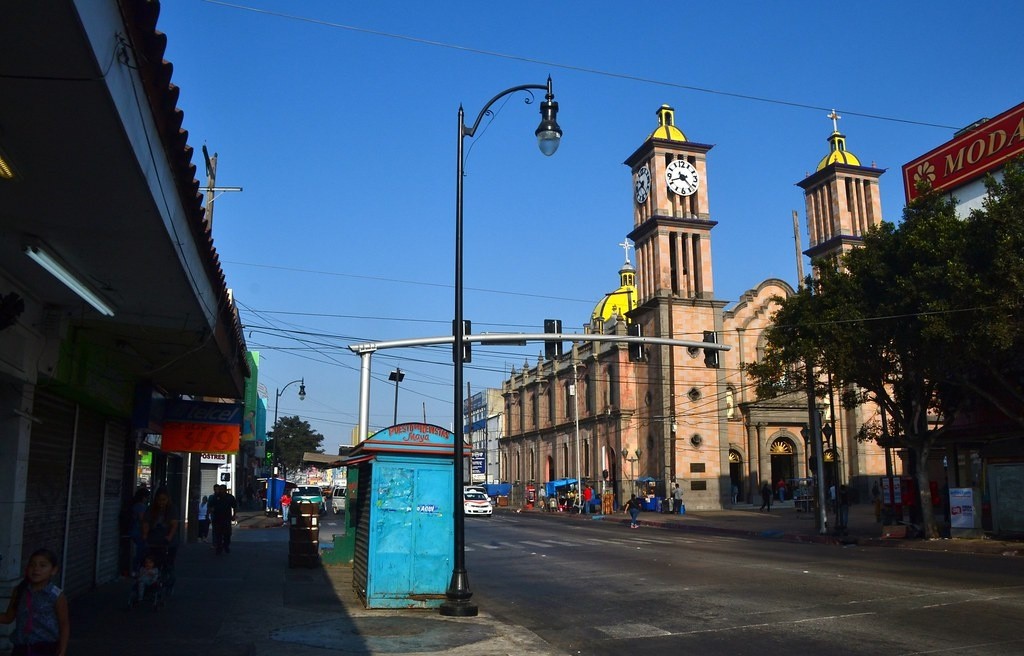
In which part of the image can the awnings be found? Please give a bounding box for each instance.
[549,478,578,489]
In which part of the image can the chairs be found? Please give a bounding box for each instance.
[550,498,558,512]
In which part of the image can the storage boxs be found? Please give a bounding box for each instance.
[289,502,321,566]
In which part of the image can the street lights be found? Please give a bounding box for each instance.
[801,421,833,528]
[388,367,405,425]
[268,377,306,516]
[622,448,643,494]
[437,74,563,616]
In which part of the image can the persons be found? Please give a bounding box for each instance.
[872,481,880,505]
[584,485,595,514]
[197,484,238,551]
[672,484,682,516]
[793,476,814,512]
[829,483,848,525]
[321,495,329,511]
[567,490,579,505]
[120,487,182,607]
[776,478,787,502]
[539,486,545,501]
[279,490,292,527]
[0,548,70,656]
[624,493,641,529]
[760,481,772,512]
[731,484,741,504]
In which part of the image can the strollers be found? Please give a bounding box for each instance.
[125,537,169,613]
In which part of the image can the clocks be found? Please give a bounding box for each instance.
[634,167,652,204]
[666,160,700,196]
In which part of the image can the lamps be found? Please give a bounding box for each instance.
[0,148,25,186]
[20,234,118,317]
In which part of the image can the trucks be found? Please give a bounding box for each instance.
[464,485,492,504]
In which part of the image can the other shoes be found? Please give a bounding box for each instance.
[216,547,221,553]
[203,538,208,543]
[631,524,638,529]
[760,508,763,511]
[223,545,229,553]
[768,509,771,511]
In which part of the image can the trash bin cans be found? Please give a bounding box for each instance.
[289,499,320,568]
[547,498,558,512]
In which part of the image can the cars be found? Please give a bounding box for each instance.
[291,484,327,517]
[463,492,493,517]
[318,479,346,514]
